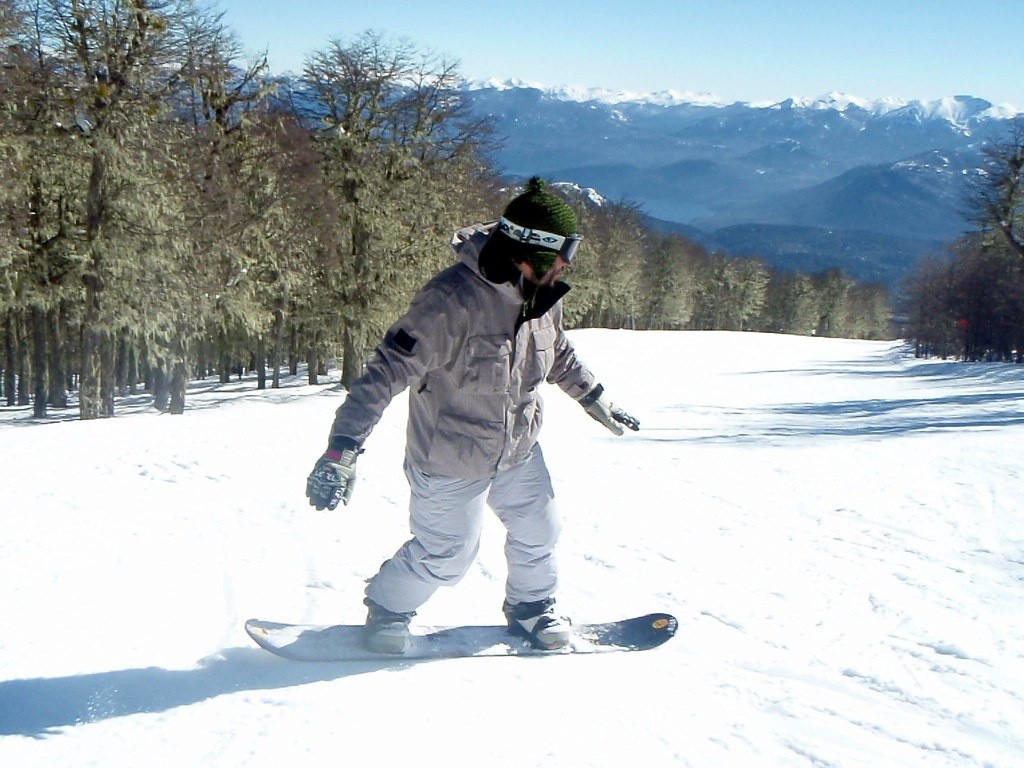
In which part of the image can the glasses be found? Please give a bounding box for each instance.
[498,216,584,265]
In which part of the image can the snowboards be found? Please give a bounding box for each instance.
[245,610,679,662]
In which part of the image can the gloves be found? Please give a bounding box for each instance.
[574,377,641,436]
[306,441,365,512]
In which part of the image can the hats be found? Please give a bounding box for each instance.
[494,175,578,280]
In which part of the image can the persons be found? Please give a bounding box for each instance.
[306,177,641,655]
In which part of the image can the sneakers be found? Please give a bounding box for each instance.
[364,612,411,654]
[502,602,571,650]
